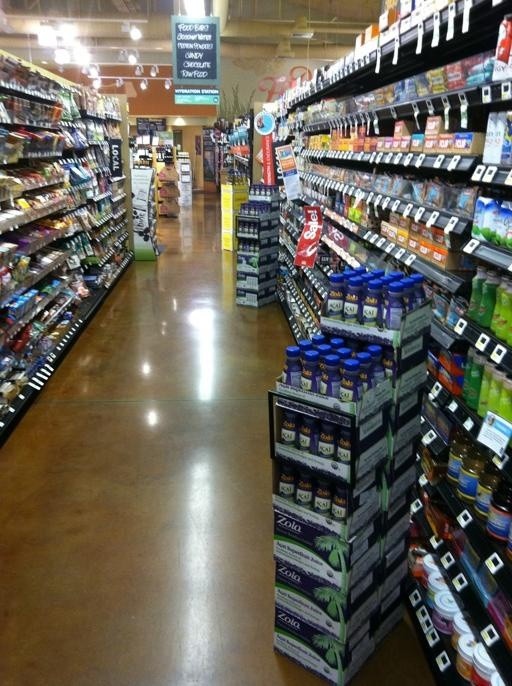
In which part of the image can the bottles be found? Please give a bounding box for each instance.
[487,371,506,414]
[357,352,372,390]
[318,420,335,458]
[496,280,512,340]
[505,526,512,561]
[499,377,512,423]
[319,355,342,398]
[299,340,312,351]
[454,447,486,505]
[281,409,297,443]
[300,350,321,392]
[381,276,393,295]
[279,465,293,497]
[322,273,343,319]
[446,430,473,485]
[382,282,406,330]
[239,203,271,216]
[474,461,503,522]
[355,267,366,275]
[297,416,315,449]
[466,265,486,319]
[372,269,385,276]
[400,277,414,313]
[466,354,484,411]
[314,476,331,512]
[337,348,351,377]
[343,276,362,322]
[339,359,362,402]
[250,184,274,196]
[318,344,332,371]
[238,239,260,253]
[477,360,495,419]
[368,345,385,387]
[330,338,344,354]
[282,345,302,387]
[389,271,404,280]
[332,481,346,517]
[312,335,326,350]
[295,469,313,504]
[237,221,258,235]
[363,280,382,326]
[490,276,510,331]
[362,271,374,290]
[409,274,425,306]
[486,479,511,543]
[476,270,498,328]
[461,347,475,401]
[336,427,351,462]
[343,269,355,291]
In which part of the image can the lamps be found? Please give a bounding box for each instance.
[35,14,173,91]
